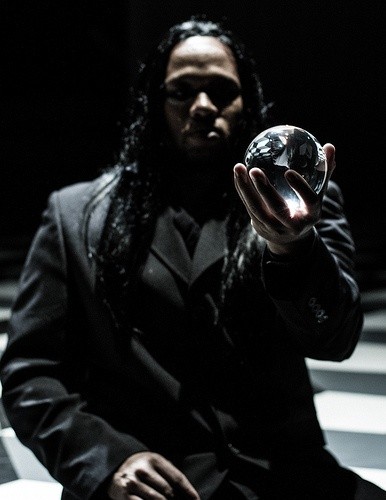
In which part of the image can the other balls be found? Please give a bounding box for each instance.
[244,124,327,208]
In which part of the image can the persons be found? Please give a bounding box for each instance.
[0,20,384,500]
